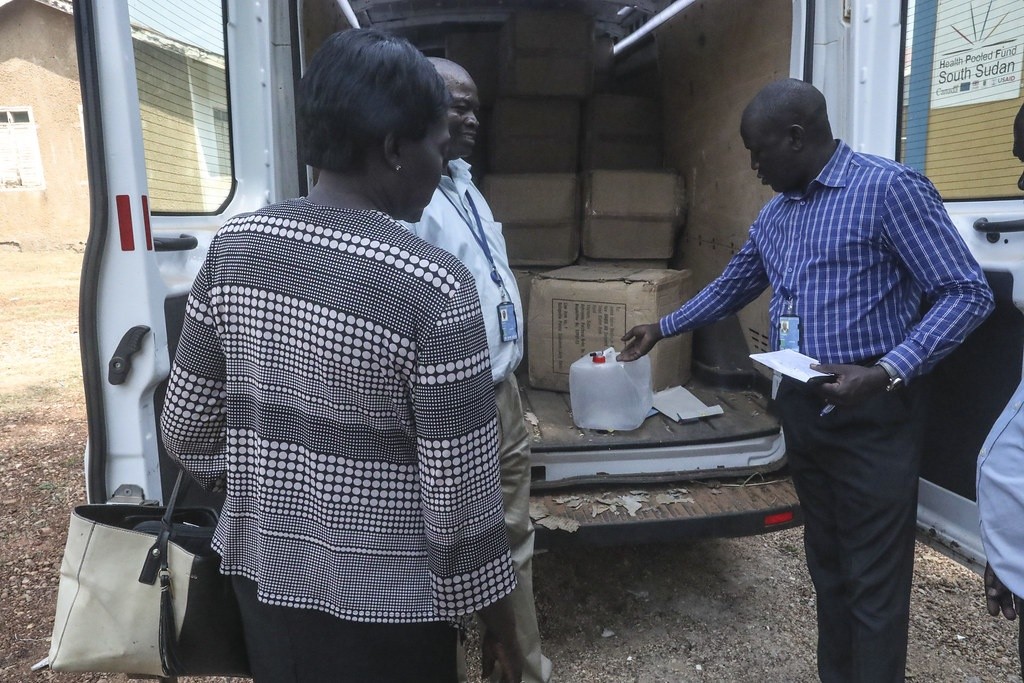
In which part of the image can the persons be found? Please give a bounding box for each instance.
[976,99,1024,683]
[395,56,553,683]
[616,78,995,683]
[160,28,522,683]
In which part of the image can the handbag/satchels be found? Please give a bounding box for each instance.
[47,469,253,679]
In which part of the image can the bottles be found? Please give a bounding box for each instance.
[566,346,654,432]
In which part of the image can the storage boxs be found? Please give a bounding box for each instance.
[447,4,695,398]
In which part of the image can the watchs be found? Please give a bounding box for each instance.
[875,361,903,394]
